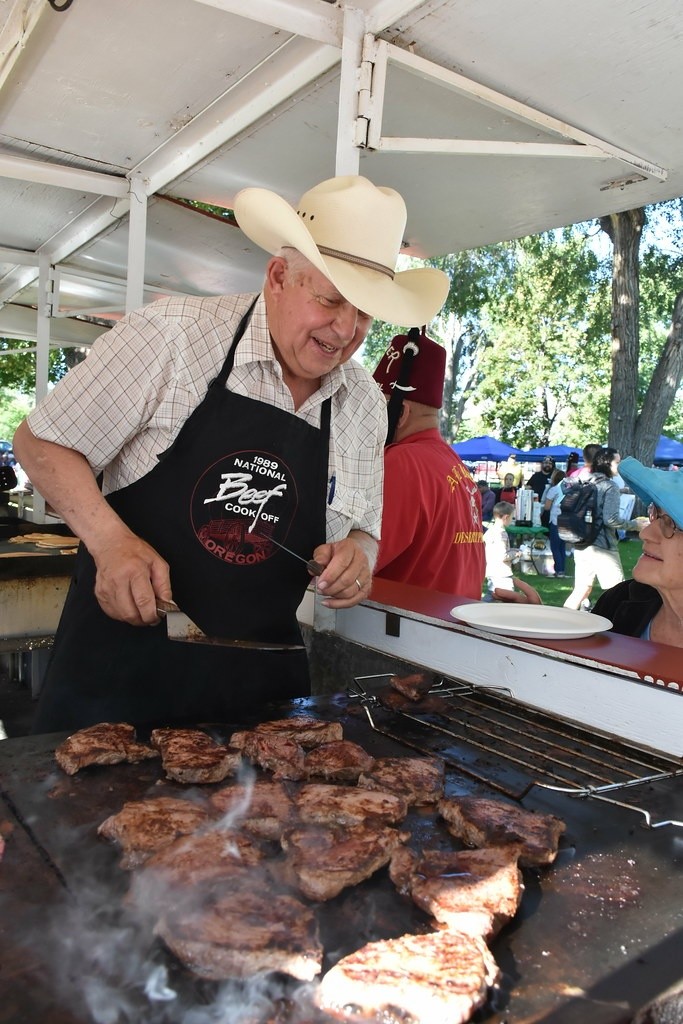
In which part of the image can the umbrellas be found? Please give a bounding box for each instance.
[653,434,683,470]
[516,444,586,463]
[448,435,523,482]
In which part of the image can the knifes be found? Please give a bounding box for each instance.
[259,532,325,577]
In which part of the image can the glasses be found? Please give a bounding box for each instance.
[647,501,683,539]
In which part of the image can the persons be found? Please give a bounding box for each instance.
[372,333,488,599]
[13,174,452,733]
[475,444,683,649]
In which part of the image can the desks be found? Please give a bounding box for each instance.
[509,526,550,577]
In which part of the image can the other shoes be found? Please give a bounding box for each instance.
[555,571,572,578]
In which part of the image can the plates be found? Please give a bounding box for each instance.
[449,603,615,638]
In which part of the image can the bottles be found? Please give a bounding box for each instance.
[585,510,592,533]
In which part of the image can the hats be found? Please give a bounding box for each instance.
[371,325,447,448]
[542,456,553,462]
[568,452,579,463]
[617,456,683,532]
[233,175,450,328]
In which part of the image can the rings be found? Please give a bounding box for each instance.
[355,579,366,591]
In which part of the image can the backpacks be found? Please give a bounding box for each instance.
[557,477,610,545]
